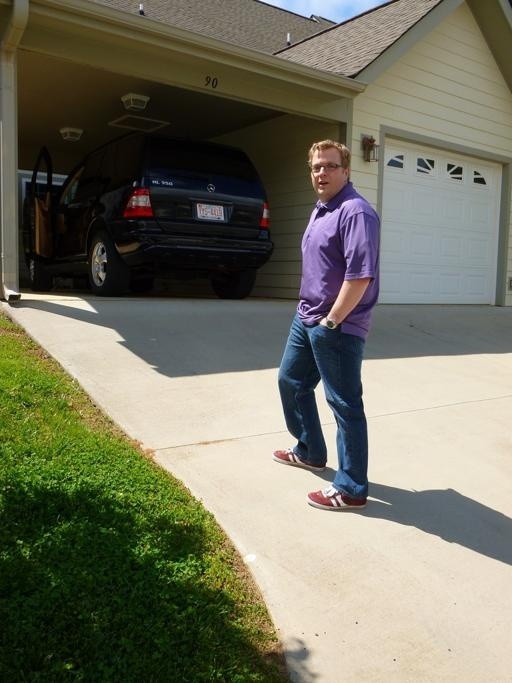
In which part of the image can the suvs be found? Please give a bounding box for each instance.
[24,128,276,299]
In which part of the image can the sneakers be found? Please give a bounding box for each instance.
[273,449,324,471]
[308,485,366,509]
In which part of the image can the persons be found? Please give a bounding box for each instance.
[272,139,379,511]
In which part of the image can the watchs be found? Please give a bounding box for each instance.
[320,315,340,328]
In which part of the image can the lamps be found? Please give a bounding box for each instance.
[359,132,378,162]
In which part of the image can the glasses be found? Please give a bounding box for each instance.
[313,163,342,171]
